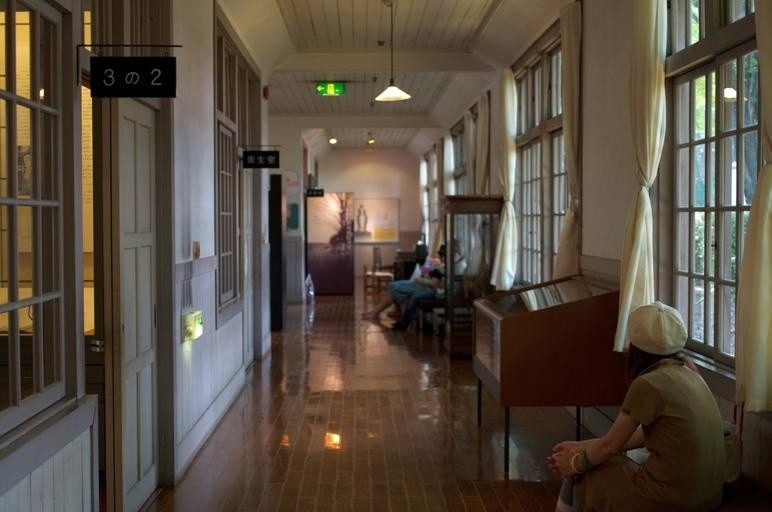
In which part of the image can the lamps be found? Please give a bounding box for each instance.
[717,87,748,105]
[367,133,375,144]
[375,0,411,102]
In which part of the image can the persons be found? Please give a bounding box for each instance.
[388,245,439,316]
[546,300,726,512]
[362,244,447,320]
[391,240,467,332]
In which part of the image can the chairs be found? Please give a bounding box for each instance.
[363,266,390,302]
[372,247,394,294]
[418,267,469,331]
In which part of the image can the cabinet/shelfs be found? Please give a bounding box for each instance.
[473,273,638,474]
[0,333,104,476]
[443,194,505,356]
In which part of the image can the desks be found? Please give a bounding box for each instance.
[395,259,419,282]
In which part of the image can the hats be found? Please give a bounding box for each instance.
[626,300,690,357]
[438,244,446,255]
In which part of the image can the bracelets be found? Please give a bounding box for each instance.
[578,447,591,470]
[570,452,584,474]
